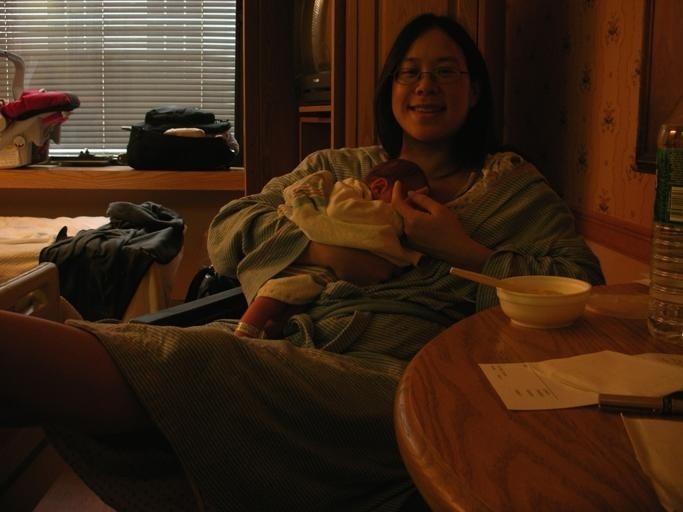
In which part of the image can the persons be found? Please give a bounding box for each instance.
[0,12,607,512]
[233,158,429,338]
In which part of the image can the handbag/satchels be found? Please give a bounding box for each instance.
[118,107,239,171]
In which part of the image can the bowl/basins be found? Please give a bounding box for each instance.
[495,274,592,330]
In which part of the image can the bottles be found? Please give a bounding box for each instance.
[648,123,683,344]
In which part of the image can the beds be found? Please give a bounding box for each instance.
[0,217,186,322]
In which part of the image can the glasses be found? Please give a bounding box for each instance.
[393,65,470,85]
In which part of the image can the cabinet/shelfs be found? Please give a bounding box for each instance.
[240,0,488,200]
